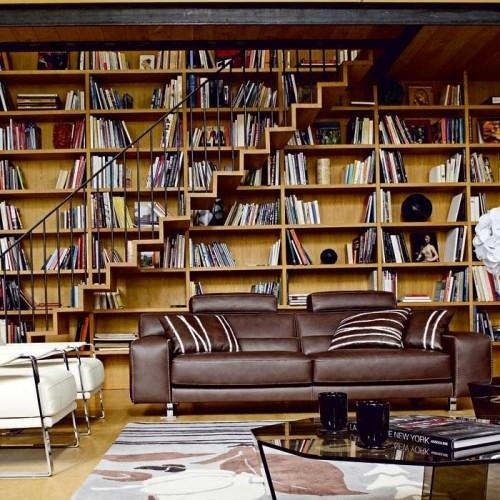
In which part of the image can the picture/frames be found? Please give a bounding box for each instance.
[406,84,435,106]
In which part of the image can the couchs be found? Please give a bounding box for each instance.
[129,290,492,420]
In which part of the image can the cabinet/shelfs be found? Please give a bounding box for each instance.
[0,48,500,392]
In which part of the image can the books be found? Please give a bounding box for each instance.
[0,49,499,353]
[347,413,500,450]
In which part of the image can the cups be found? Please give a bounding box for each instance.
[317,393,348,434]
[355,400,394,450]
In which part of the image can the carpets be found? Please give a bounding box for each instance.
[70,420,500,500]
[469,377,500,424]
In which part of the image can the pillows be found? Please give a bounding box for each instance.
[328,306,415,352]
[403,309,456,350]
[157,313,242,355]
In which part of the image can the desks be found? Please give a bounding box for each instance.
[250,414,500,499]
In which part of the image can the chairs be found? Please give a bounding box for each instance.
[0,333,105,436]
[0,348,81,477]
